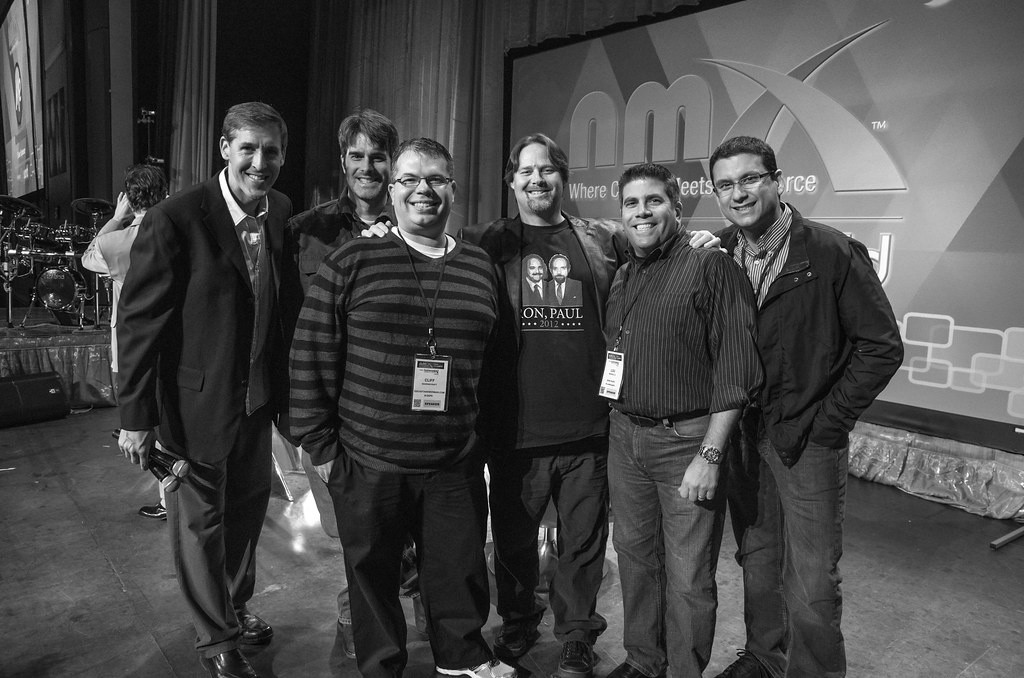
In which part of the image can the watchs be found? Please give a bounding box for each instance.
[698,445,723,464]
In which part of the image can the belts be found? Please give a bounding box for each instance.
[623,407,711,427]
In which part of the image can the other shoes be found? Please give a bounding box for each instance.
[414,596,426,635]
[337,619,355,659]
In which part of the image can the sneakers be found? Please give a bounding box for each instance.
[437,656,515,678]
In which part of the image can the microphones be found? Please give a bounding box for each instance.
[112,429,192,479]
[149,460,181,491]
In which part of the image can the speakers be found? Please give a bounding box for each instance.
[0,371,70,428]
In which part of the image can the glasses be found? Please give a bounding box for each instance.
[393,175,453,186]
[716,170,775,194]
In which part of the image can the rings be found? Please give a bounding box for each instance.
[698,496,705,500]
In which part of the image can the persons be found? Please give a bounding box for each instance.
[521,254,549,305]
[547,253,583,304]
[709,136,903,677]
[284,137,518,678]
[112,101,296,678]
[81,164,168,519]
[602,164,766,676]
[355,132,728,677]
[284,110,432,678]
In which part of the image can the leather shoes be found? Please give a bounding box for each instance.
[604,663,646,678]
[559,642,593,678]
[715,651,773,678]
[200,649,257,678]
[234,611,274,642]
[139,502,167,520]
[494,621,536,657]
